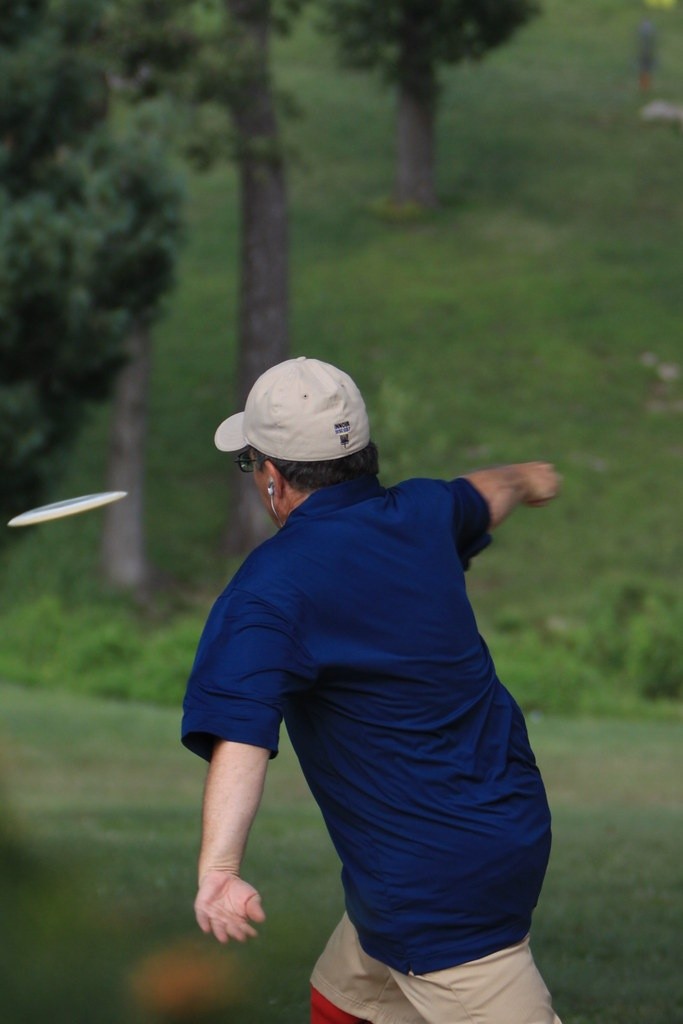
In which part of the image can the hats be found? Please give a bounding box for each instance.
[215,356,370,462]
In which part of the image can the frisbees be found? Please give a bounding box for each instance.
[6,490,128,527]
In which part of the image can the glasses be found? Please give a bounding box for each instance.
[235,450,258,473]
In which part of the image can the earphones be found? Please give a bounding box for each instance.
[267,477,275,496]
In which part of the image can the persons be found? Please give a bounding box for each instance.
[181,357,561,1024]
